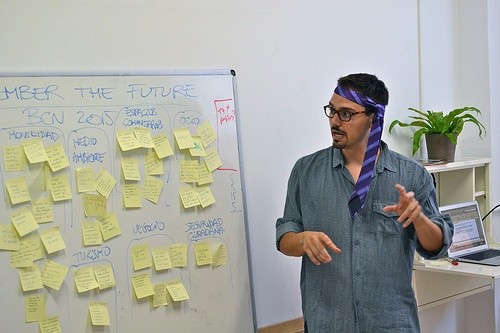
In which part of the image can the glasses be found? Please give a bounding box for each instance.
[323,104,368,123]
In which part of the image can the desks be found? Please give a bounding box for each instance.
[412,261,500,333]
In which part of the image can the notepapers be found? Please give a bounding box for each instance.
[431,261,448,266]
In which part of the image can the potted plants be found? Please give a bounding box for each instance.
[389,106,487,162]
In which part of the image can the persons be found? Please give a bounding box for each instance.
[275,73,454,333]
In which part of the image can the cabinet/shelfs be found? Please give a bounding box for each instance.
[413,163,491,242]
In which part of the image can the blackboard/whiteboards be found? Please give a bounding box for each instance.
[0,70,258,333]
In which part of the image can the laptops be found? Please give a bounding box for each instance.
[436,200,500,266]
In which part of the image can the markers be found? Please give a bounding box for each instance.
[446,257,458,265]
